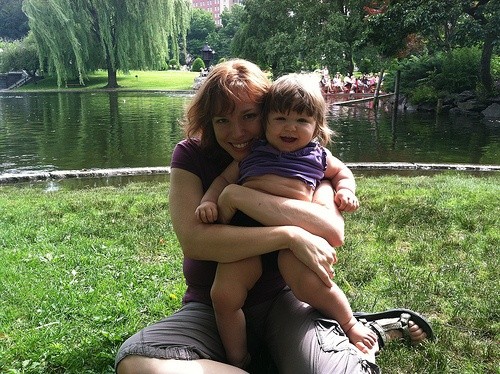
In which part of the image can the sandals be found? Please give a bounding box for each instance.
[341,309,435,350]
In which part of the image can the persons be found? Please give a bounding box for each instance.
[311,63,387,97]
[114,59,435,373]
[193,72,379,369]
